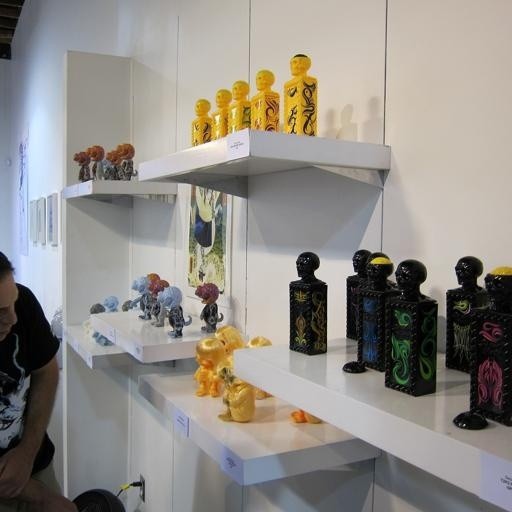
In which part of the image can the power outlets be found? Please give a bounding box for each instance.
[139,473,145,502]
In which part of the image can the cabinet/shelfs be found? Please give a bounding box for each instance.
[68,128,512,511]
[29,192,58,247]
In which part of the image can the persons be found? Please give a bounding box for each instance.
[0,251,82,510]
